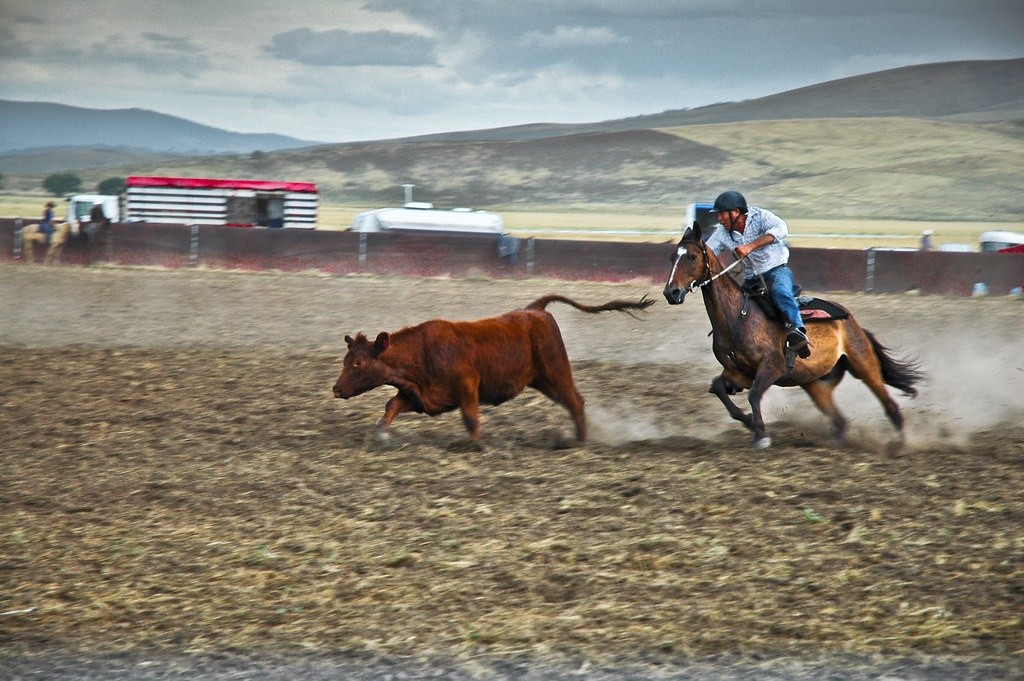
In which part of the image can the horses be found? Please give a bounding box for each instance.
[14,218,102,267]
[661,219,930,451]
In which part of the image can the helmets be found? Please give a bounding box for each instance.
[709,191,748,215]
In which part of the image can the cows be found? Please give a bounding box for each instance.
[332,293,657,443]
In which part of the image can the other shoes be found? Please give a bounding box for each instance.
[787,331,811,359]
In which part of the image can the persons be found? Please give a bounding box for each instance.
[922,231,934,250]
[43,202,55,244]
[91,202,105,222]
[705,191,812,359]
